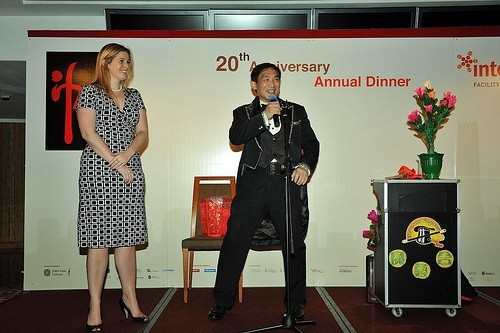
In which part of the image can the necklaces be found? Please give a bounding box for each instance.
[111,84,122,92]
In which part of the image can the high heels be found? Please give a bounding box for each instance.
[120,297,148,323]
[85,305,103,333]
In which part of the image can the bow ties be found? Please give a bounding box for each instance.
[261,104,268,111]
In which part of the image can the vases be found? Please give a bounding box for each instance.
[417,153,445,180]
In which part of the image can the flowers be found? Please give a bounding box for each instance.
[361,209,379,239]
[403,78,457,153]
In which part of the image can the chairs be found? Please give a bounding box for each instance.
[180,173,245,305]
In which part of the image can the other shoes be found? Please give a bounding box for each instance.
[208,304,226,320]
[290,305,305,320]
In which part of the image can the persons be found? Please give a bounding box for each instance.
[75,43,150,333]
[209,62,320,324]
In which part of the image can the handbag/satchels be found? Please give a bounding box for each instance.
[199,196,233,237]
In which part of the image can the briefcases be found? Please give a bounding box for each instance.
[366,254,379,304]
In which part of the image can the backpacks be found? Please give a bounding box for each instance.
[460,268,477,303]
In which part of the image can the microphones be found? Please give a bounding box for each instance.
[267,95,280,127]
